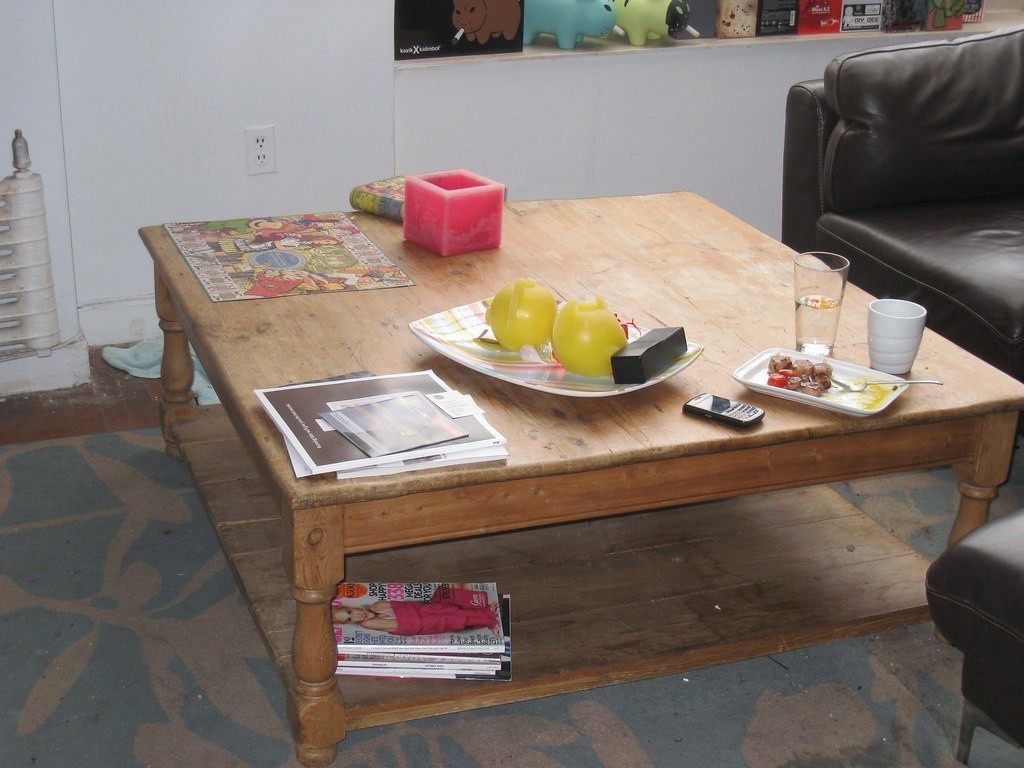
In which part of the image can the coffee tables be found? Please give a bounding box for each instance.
[140,191,1024,768]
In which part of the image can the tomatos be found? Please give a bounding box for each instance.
[767,369,798,387]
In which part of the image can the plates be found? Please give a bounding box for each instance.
[732,348,910,417]
[407,296,705,398]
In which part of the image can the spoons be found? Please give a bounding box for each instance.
[829,375,944,390]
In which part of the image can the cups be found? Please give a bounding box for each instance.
[793,251,850,357]
[868,299,927,374]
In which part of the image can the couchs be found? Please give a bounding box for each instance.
[925,508,1024,768]
[781,24,1023,383]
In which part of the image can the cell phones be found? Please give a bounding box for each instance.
[683,393,765,429]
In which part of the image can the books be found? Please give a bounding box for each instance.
[254,369,510,482]
[328,579,513,684]
[349,173,408,222]
